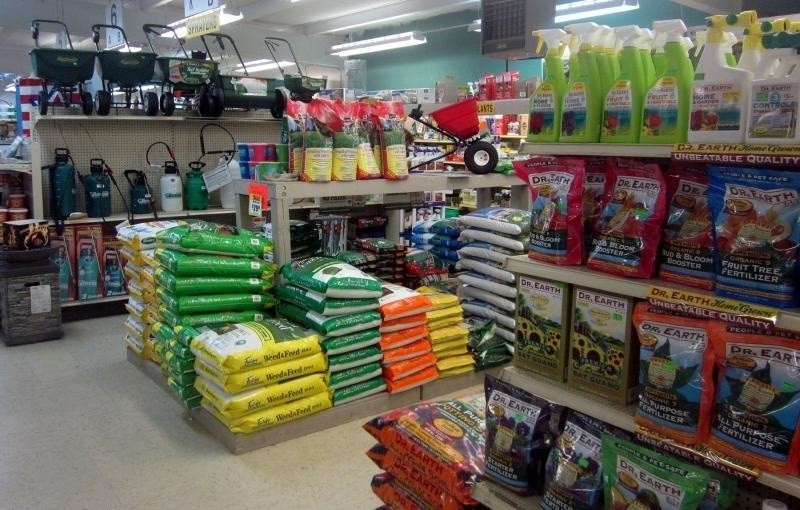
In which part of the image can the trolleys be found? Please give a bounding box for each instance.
[408,96,497,174]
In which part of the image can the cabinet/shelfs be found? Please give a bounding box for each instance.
[460,140,800,509]
[0,104,534,298]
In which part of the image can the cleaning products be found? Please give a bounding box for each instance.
[527,11,799,145]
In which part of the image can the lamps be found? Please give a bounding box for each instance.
[468,1,642,40]
[329,28,430,61]
[107,9,295,97]
